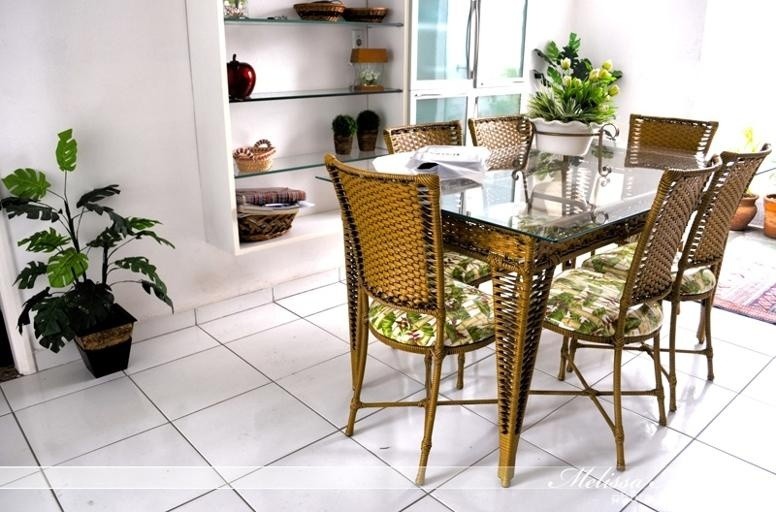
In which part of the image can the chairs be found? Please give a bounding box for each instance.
[325,111,773,489]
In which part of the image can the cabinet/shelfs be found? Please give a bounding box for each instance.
[225,15,402,180]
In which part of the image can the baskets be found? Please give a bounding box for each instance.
[237,204,300,241]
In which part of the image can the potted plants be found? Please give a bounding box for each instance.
[0,127,179,377]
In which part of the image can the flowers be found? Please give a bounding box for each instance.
[520,55,623,127]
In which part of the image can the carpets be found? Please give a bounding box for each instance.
[706,237,776,324]
